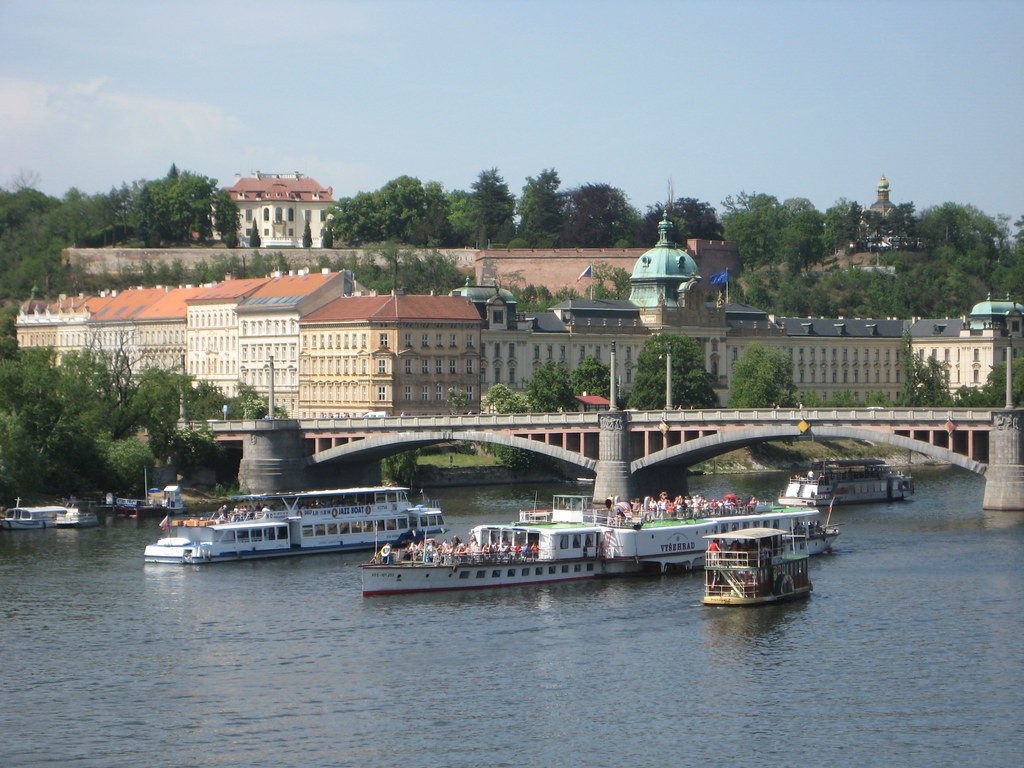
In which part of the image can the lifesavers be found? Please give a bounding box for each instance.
[380,545,393,559]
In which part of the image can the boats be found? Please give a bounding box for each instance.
[51,503,100,528]
[777,455,916,507]
[0,496,67,530]
[161,485,189,514]
[143,485,450,566]
[355,489,846,599]
[699,517,815,606]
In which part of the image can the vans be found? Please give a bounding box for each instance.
[364,410,388,418]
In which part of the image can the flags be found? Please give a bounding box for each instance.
[577,265,591,282]
[159,515,169,531]
[711,270,728,284]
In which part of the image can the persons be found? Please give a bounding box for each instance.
[795,467,853,485]
[605,491,822,591]
[219,495,366,523]
[403,535,540,566]
